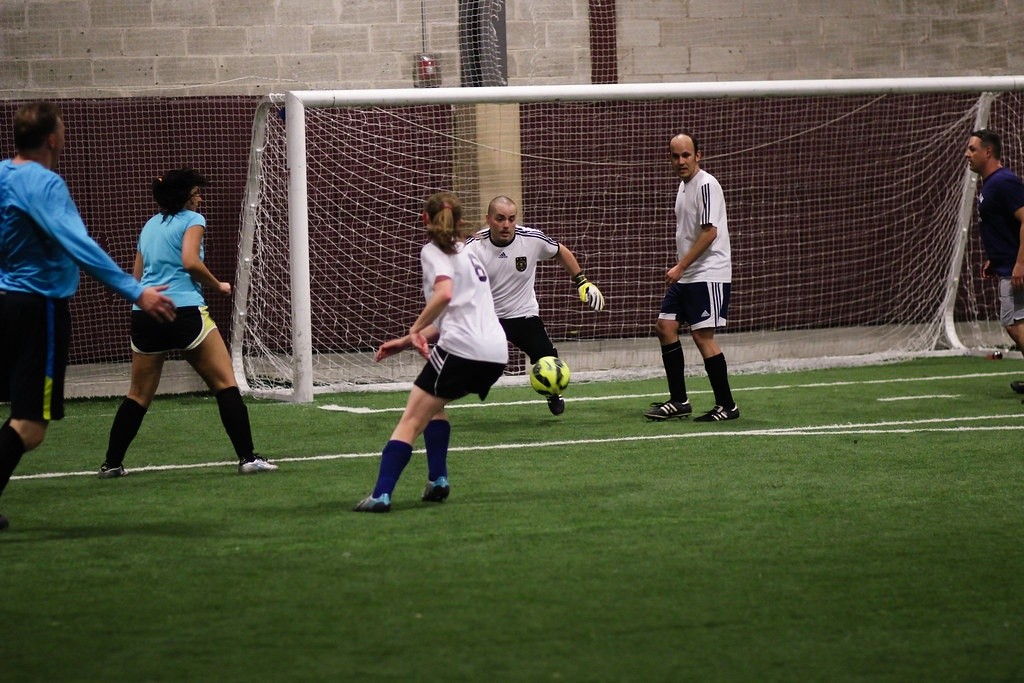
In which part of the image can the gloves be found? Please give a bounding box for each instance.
[573,271,604,312]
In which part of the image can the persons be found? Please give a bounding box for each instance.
[643,132,740,422]
[0,99,179,530]
[353,194,509,513]
[462,196,605,415]
[964,129,1024,404]
[98,170,280,479]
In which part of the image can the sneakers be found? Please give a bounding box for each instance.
[422,476,450,502]
[353,493,391,512]
[238,453,278,475]
[693,403,739,422]
[644,400,692,421]
[98,463,128,479]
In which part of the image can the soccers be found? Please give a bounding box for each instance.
[530,356,571,398]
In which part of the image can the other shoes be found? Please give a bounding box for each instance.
[546,394,565,415]
[1010,380,1024,394]
